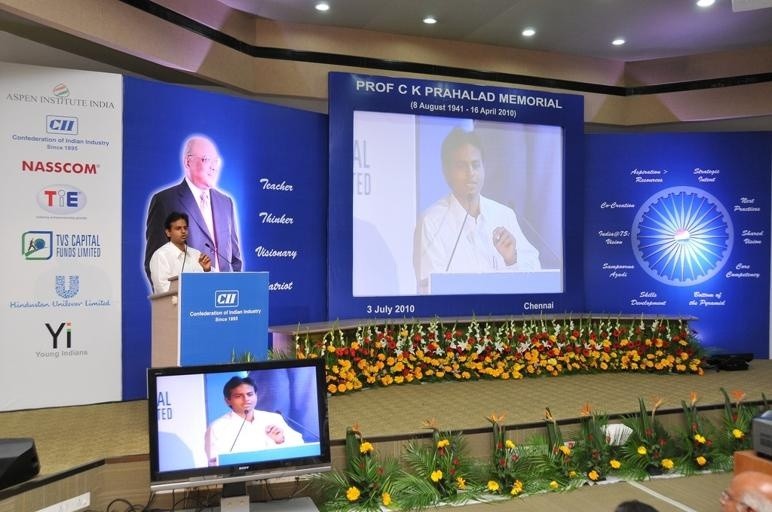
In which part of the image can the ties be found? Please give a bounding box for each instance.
[200,191,208,210]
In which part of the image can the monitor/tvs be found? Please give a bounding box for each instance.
[146,357,332,512]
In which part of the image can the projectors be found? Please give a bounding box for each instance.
[750,410,772,457]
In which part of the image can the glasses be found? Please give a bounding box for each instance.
[188,155,220,165]
[721,488,745,506]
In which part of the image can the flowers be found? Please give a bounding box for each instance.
[588,470,599,480]
[437,439,449,447]
[661,458,674,468]
[275,310,704,391]
[350,422,374,454]
[505,439,516,450]
[457,477,466,488]
[510,480,523,495]
[732,428,745,438]
[346,486,360,500]
[609,459,621,469]
[382,492,391,505]
[695,456,706,465]
[695,434,707,443]
[486,481,499,490]
[637,445,647,454]
[550,481,558,489]
[559,445,570,455]
[431,470,443,482]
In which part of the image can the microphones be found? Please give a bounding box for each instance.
[276,410,319,439]
[507,200,560,260]
[446,194,475,272]
[204,242,240,272]
[182,239,188,272]
[229,409,249,453]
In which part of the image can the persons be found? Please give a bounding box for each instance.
[614,469,772,511]
[204,377,305,468]
[144,133,243,294]
[412,128,542,295]
[149,212,220,295]
